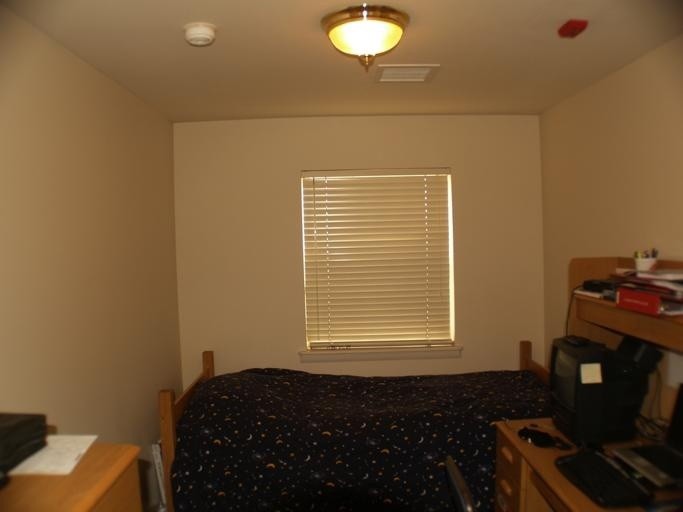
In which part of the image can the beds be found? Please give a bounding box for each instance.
[159,340,548,512]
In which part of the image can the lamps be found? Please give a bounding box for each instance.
[320,1,411,73]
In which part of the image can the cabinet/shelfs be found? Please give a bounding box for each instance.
[492,257,683,512]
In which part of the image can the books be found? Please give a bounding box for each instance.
[615,268,683,316]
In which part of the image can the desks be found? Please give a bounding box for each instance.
[1,434,144,511]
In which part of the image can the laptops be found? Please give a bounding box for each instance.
[611,383,683,489]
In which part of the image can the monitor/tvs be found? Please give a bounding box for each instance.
[548,335,649,445]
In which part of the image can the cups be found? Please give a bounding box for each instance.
[635,258,658,273]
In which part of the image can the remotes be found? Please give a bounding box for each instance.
[561,335,591,347]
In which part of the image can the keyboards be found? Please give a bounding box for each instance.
[555,451,650,508]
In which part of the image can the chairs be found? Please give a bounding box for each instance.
[444,455,477,512]
